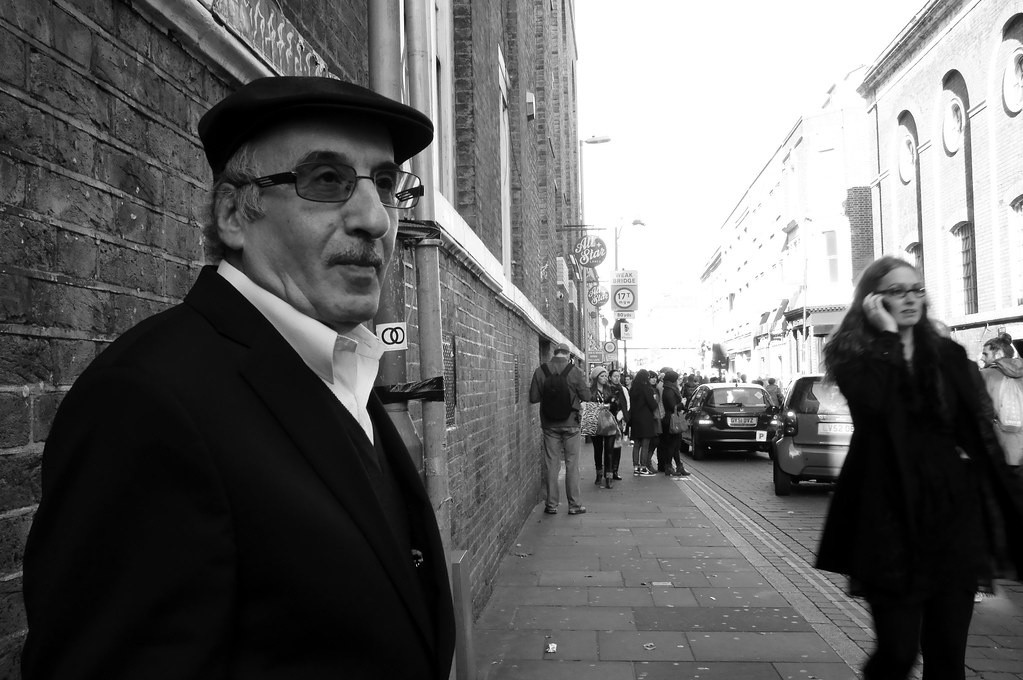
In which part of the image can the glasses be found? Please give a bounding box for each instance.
[253,161,424,210]
[877,286,926,299]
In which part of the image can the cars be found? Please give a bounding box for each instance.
[680,382,775,459]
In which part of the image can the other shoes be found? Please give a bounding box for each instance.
[568,507,586,514]
[545,506,557,514]
[633,465,656,477]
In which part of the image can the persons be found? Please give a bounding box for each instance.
[16,76,458,680]
[529,343,592,515]
[590,366,784,489]
[972,332,1023,602]
[812,255,1012,680]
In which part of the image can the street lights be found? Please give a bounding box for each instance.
[580,136,612,385]
[615,220,648,366]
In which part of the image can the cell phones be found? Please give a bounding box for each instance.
[882,299,891,313]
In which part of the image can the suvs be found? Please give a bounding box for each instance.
[767,375,856,496]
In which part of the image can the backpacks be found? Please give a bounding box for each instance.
[997,376,1022,432]
[540,362,575,423]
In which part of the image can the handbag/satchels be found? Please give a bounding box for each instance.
[580,402,600,436]
[596,404,617,436]
[669,405,689,434]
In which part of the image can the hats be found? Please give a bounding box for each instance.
[554,343,569,352]
[197,75,434,178]
[590,366,606,380]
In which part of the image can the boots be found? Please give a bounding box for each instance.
[613,465,622,480]
[595,468,603,485]
[676,463,690,476]
[605,472,614,489]
[665,463,681,476]
